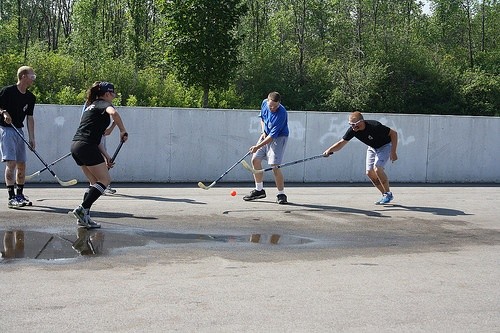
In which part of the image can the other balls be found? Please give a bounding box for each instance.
[231,191,236,196]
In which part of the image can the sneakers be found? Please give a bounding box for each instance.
[8,197,25,207]
[77,215,101,227]
[15,195,32,206]
[374,196,384,205]
[73,205,90,229]
[243,189,266,200]
[380,192,394,204]
[276,194,287,203]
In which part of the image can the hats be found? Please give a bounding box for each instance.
[98,82,114,93]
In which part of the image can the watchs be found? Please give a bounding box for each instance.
[2,110,6,115]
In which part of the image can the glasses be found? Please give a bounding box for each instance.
[24,75,36,80]
[349,120,363,126]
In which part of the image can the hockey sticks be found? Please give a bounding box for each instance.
[25,151,71,180]
[240,151,334,173]
[10,122,77,187]
[107,133,129,169]
[198,150,253,190]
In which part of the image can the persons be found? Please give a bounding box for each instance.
[72,228,104,256]
[323,112,397,204]
[71,81,128,227]
[0,66,37,206]
[249,234,280,243]
[4,231,25,259]
[243,92,289,204]
